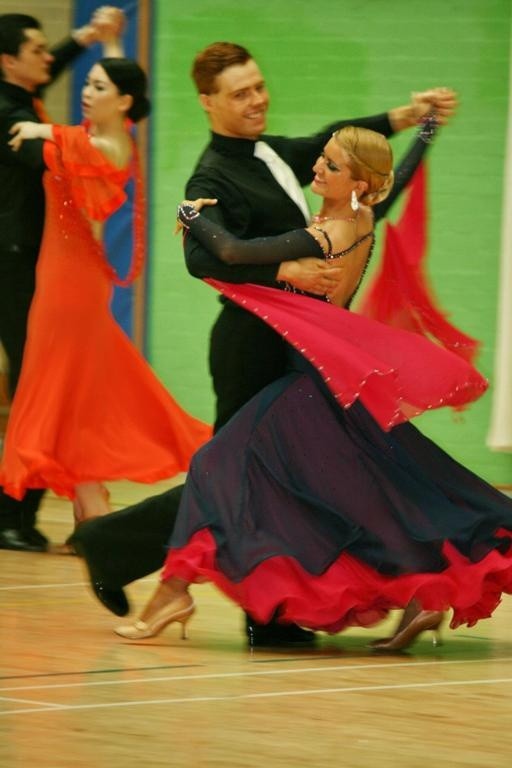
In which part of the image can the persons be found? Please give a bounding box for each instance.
[114,88,453,654]
[0,8,214,547]
[71,42,456,648]
[2,5,126,552]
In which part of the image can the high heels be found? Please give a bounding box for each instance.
[111,592,197,640]
[363,609,443,651]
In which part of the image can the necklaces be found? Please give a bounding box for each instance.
[314,208,361,225]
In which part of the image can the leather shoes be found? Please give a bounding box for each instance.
[71,519,129,616]
[247,619,316,646]
[0,499,49,552]
